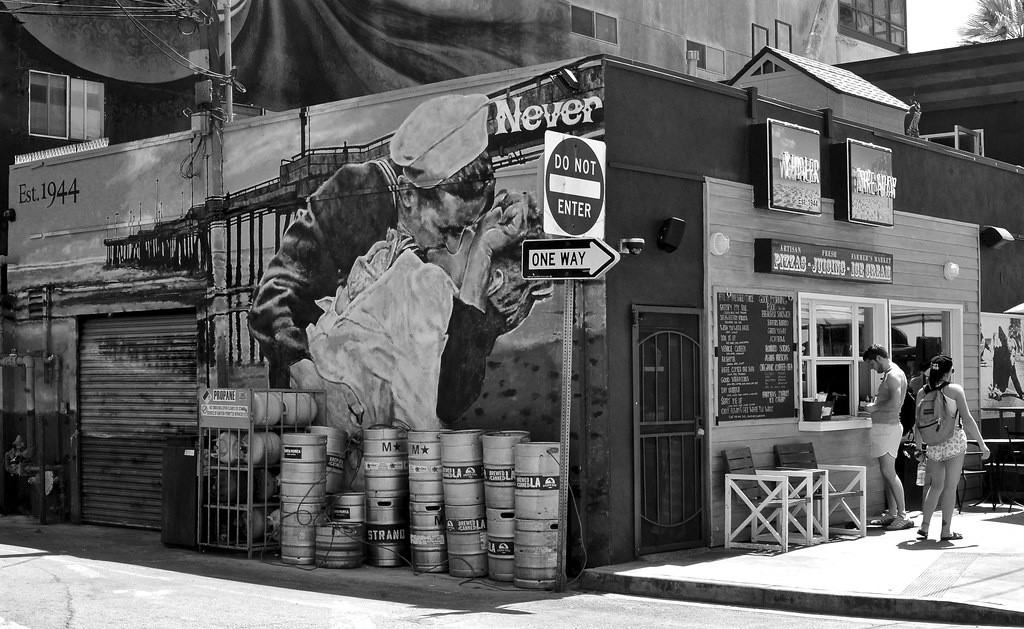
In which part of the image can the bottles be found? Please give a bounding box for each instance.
[915,460,926,487]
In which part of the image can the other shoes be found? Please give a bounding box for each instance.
[870,513,895,525]
[885,515,914,530]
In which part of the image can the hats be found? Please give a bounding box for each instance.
[924,355,952,377]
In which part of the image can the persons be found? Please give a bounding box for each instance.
[915,356,991,540]
[284,195,555,431]
[863,344,915,531]
[248,93,527,430]
[989,337,1024,400]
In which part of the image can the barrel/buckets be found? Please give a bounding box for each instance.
[279,421,560,588]
[804,401,835,421]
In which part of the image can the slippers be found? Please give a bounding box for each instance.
[941,532,963,540]
[917,529,928,537]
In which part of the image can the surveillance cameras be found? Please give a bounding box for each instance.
[4,208,17,222]
[628,238,643,255]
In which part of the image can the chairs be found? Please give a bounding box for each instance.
[721,440,867,554]
[956,461,988,515]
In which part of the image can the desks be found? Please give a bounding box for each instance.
[967,438,1024,512]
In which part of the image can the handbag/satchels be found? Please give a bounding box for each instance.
[900,387,916,436]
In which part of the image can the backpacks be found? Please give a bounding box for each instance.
[915,380,958,446]
